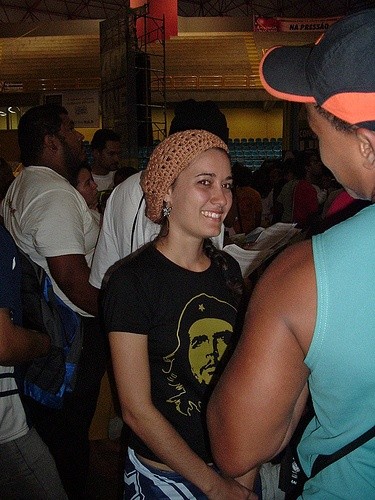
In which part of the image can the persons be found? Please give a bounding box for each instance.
[0,103,359,500]
[206,9,375,500]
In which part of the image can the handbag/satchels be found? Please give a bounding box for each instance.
[277,453,304,495]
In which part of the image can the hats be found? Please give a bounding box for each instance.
[170,99,227,133]
[261,11,375,131]
[140,130,229,223]
